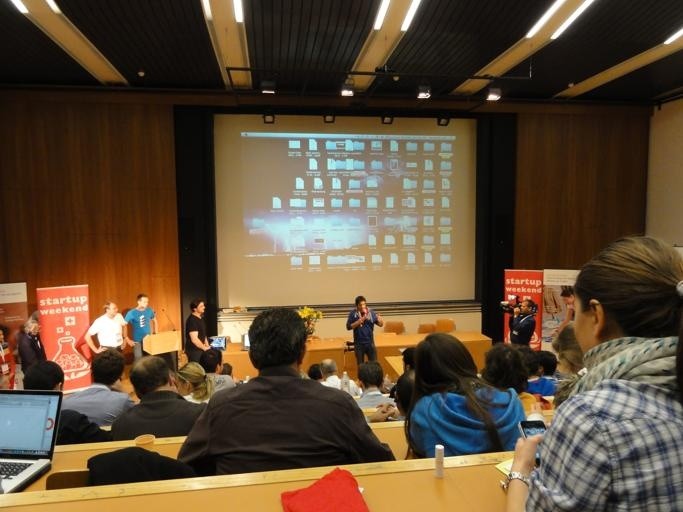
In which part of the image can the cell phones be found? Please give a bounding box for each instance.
[517,420,547,465]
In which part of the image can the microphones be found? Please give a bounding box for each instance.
[359,312,367,329]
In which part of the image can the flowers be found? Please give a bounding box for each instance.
[295,304,324,333]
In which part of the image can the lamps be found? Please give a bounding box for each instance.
[259,69,502,102]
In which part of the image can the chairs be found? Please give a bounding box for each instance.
[418,322,436,333]
[382,321,407,335]
[435,318,457,333]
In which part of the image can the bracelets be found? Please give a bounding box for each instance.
[507,471,529,486]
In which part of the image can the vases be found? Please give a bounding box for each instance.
[305,326,317,339]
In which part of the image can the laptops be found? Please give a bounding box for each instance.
[1,391,63,495]
[208,336,226,352]
[240,333,251,352]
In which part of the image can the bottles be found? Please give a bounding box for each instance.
[340,371,350,396]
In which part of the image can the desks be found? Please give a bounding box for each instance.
[181,328,493,385]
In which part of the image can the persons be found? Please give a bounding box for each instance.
[1,288,583,476]
[506,235,683,511]
